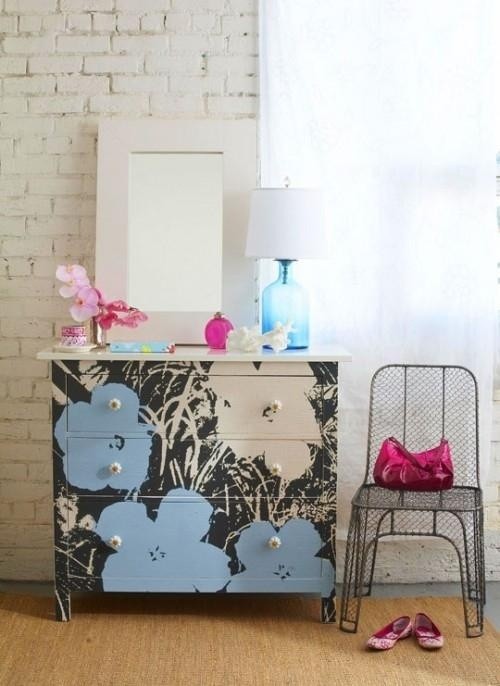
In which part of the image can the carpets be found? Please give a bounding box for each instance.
[1,592,500,685]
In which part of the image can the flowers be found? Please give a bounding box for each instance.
[55,258,149,340]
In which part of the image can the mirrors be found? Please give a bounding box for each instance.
[93,118,246,348]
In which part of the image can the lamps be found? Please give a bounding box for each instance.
[243,183,332,349]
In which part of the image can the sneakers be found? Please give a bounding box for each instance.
[414,613,444,649]
[367,616,412,650]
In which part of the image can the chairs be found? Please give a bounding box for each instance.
[338,363,490,636]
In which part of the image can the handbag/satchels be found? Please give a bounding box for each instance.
[374,437,454,490]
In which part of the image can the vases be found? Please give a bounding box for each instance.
[87,315,109,352]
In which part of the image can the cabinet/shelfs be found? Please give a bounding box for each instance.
[38,337,353,625]
